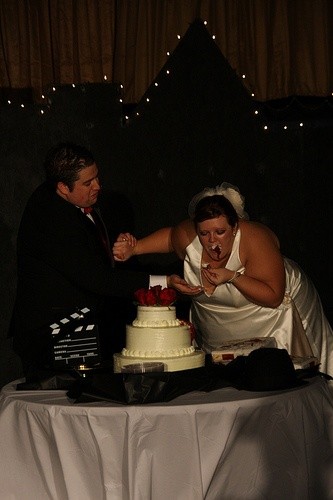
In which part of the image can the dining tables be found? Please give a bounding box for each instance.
[1,376,333,500]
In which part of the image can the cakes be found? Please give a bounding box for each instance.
[121,284,194,357]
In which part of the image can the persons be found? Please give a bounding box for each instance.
[112,182,331,379]
[20,152,135,390]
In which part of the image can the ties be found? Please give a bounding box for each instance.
[84,206,111,256]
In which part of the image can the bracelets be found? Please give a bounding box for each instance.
[228,271,242,284]
